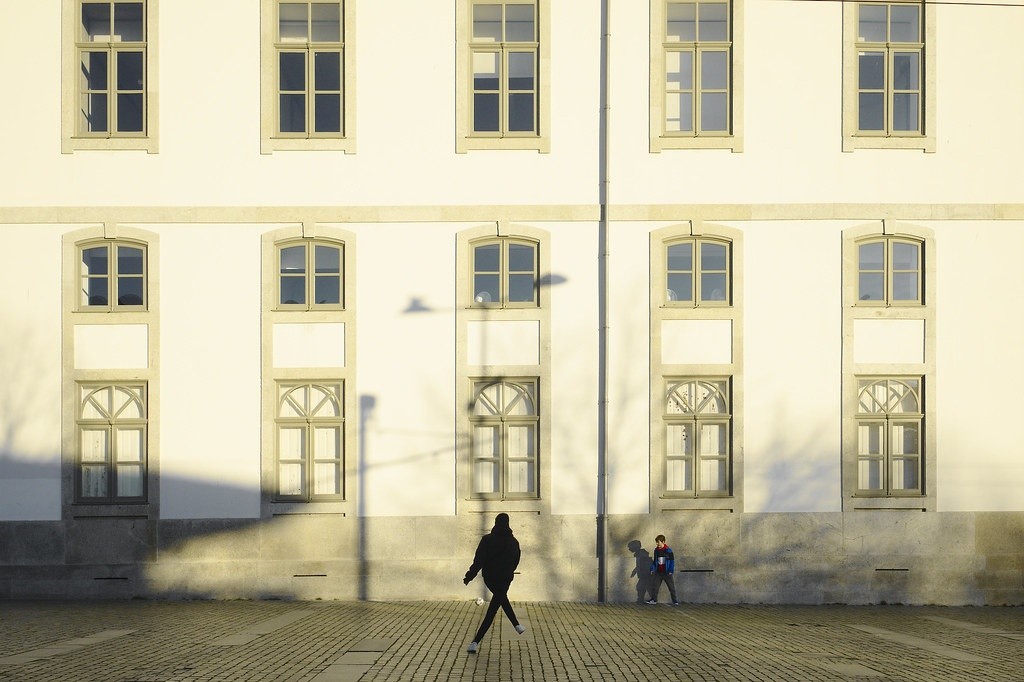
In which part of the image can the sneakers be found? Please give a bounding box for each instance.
[468,642,478,652]
[515,624,525,634]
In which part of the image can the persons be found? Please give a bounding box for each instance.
[644,535,678,606]
[463,512,525,652]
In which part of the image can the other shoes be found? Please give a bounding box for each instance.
[646,599,657,604]
[673,601,679,605]
